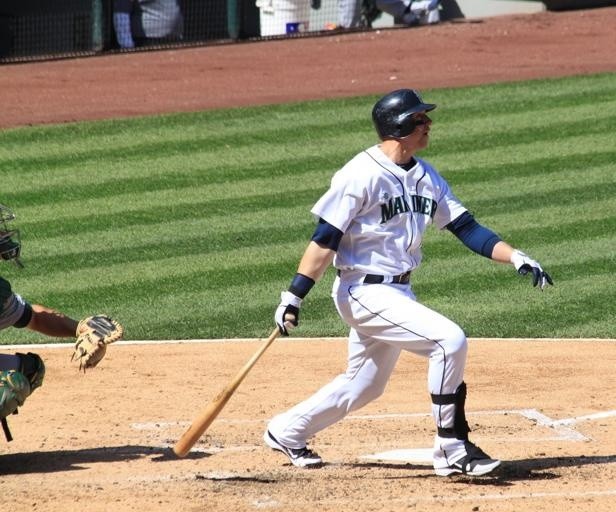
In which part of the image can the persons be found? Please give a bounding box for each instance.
[111,1,182,48]
[262,88,555,475]
[0,204,122,424]
[337,0,439,29]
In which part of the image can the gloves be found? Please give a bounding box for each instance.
[274,272,315,336]
[511,248,553,292]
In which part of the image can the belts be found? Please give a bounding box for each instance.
[336,269,410,284]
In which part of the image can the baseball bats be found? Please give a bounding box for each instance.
[173,313,295,458]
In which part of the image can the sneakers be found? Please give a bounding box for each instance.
[264,428,322,468]
[434,453,502,476]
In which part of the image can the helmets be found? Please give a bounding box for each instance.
[372,88,436,139]
[0,204,23,260]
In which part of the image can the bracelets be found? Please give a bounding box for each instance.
[288,273,315,299]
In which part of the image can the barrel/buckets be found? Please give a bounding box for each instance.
[256,1,309,35]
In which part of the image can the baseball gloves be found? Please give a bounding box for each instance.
[71,314,122,374]
